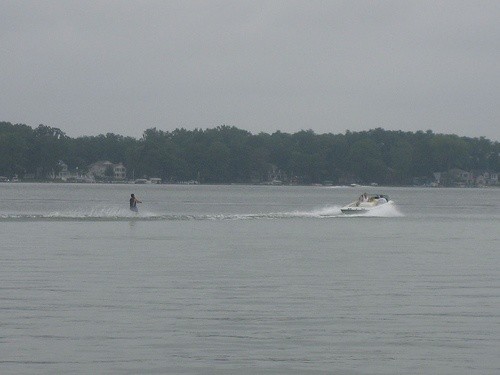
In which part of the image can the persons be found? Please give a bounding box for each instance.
[361,192,370,202]
[129,193,139,213]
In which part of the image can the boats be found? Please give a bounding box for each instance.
[340,192,393,214]
